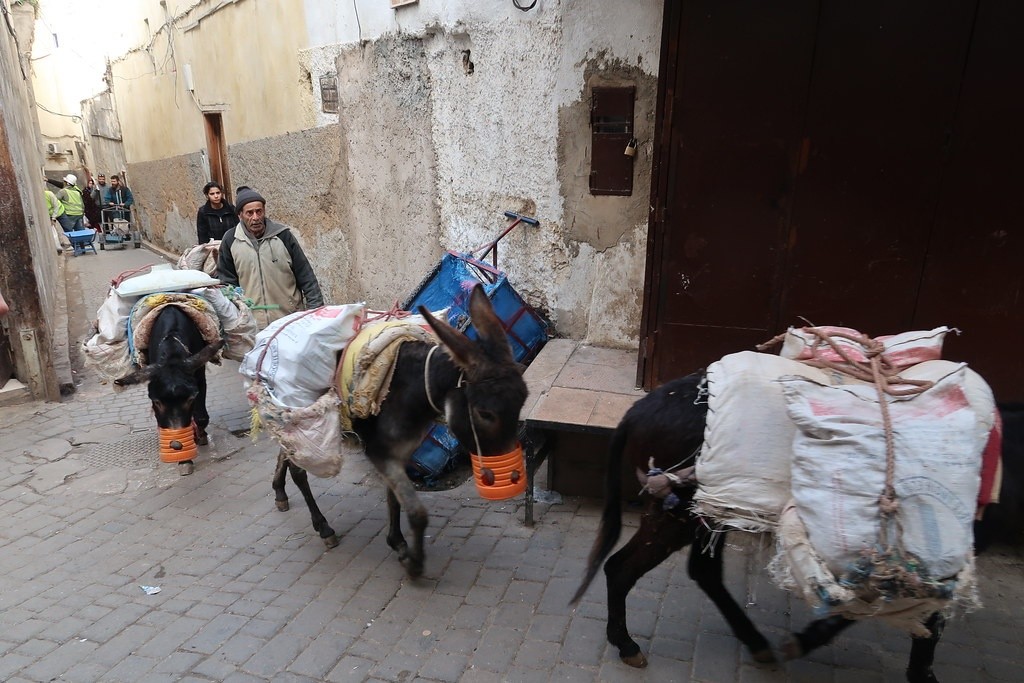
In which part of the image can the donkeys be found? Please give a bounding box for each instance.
[565,354,953,683]
[113,304,228,477]
[273,283,529,578]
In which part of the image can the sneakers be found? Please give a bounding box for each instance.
[65,245,74,251]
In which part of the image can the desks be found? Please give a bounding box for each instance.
[517,334,650,527]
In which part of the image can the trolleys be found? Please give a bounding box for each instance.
[61,228,98,257]
[402,211,551,490]
[98,204,142,250]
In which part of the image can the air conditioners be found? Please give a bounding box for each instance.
[47,142,62,154]
[45,142,63,155]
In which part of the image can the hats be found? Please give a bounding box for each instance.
[235,185,267,214]
[63,174,78,186]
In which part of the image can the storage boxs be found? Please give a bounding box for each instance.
[400,249,550,475]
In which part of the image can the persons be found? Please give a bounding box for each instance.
[89,173,113,234]
[44,190,75,251]
[43,176,64,189]
[55,173,84,232]
[104,174,132,231]
[215,184,324,336]
[196,181,239,245]
[81,179,99,229]
